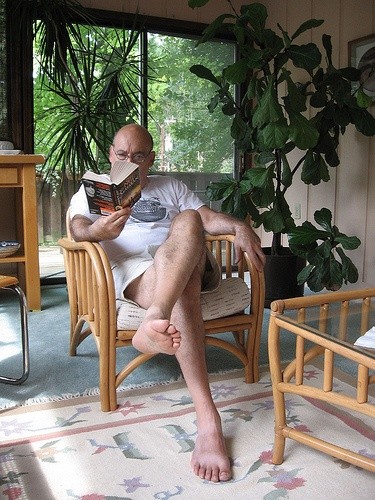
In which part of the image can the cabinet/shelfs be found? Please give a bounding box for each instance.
[0,155,45,312]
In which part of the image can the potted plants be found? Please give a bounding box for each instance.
[185,0,375,309]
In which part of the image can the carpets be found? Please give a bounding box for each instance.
[0,357,375,499]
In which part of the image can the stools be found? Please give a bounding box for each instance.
[0,276,30,384]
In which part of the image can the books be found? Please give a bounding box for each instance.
[81,160,142,216]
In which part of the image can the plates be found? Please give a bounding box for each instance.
[0,150,21,155]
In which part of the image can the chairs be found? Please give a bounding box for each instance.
[58,207,266,412]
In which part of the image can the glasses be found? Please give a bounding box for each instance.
[111,144,152,164]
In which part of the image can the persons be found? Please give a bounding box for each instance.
[70,125,267,482]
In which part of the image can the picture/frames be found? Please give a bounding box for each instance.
[348,33,375,105]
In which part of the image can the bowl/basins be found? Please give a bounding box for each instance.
[0,141,14,150]
[0,241,21,257]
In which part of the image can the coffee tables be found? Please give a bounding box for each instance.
[267,289,375,473]
